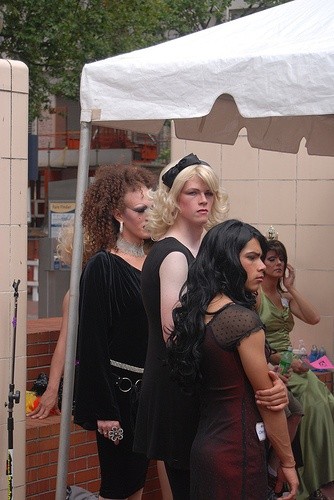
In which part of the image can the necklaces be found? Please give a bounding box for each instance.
[115,234,145,257]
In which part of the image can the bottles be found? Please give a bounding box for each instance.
[276,346,295,380]
[157,120,171,163]
[292,338,328,374]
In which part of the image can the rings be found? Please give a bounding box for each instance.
[109,427,124,441]
[104,432,107,434]
[98,428,102,432]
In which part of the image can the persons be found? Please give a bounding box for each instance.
[26,154,230,500]
[162,219,300,500]
[255,240,334,500]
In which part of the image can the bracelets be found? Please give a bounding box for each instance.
[280,463,296,468]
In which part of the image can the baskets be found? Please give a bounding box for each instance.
[67,139,80,150]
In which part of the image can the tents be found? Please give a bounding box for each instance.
[55,0,334,500]
